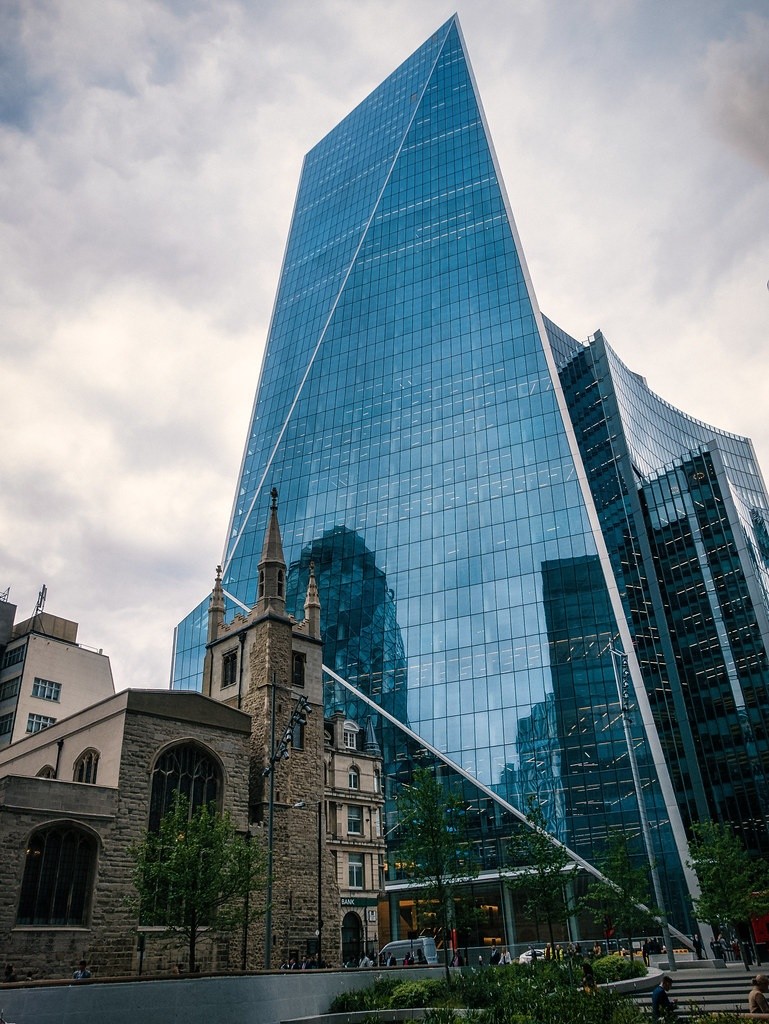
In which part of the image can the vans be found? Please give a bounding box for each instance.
[379,937,439,965]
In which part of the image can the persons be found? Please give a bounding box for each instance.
[581,963,594,992]
[749,975,769,1014]
[171,962,179,975]
[72,960,91,979]
[489,944,512,965]
[344,952,397,968]
[526,941,601,963]
[652,976,678,1020]
[279,952,333,969]
[693,934,742,963]
[403,949,428,964]
[643,937,667,967]
[452,949,464,966]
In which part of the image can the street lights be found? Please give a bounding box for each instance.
[261,670,312,974]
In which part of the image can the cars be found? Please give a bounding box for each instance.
[518,949,552,963]
